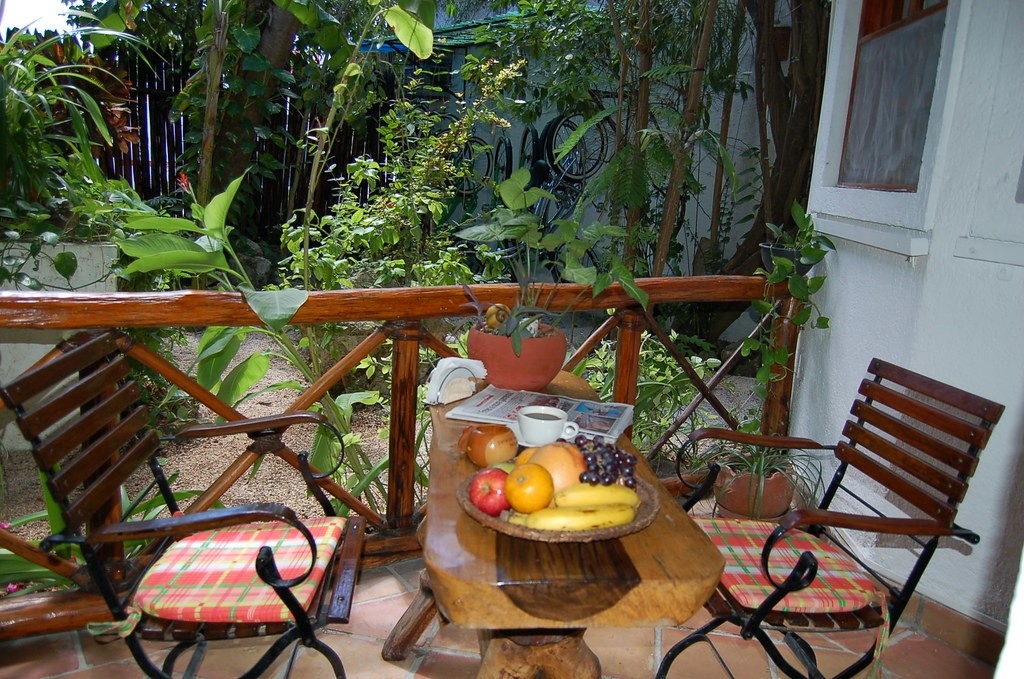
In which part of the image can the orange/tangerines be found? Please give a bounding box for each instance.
[504,463,554,514]
[514,448,538,467]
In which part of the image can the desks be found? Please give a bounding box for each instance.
[381,371,727,679]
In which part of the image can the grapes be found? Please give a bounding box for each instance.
[557,436,636,490]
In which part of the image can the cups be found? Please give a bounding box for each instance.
[517,405,579,447]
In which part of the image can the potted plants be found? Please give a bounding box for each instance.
[701,434,828,520]
[730,198,837,436]
[447,168,649,392]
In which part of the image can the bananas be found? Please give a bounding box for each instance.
[508,482,640,532]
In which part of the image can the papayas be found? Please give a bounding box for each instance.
[529,444,576,509]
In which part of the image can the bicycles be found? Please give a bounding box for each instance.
[442,108,610,284]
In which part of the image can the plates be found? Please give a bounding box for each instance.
[506,423,575,447]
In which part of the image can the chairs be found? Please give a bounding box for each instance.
[0,328,367,679]
[654,358,1005,679]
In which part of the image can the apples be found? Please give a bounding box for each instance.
[470,469,511,517]
